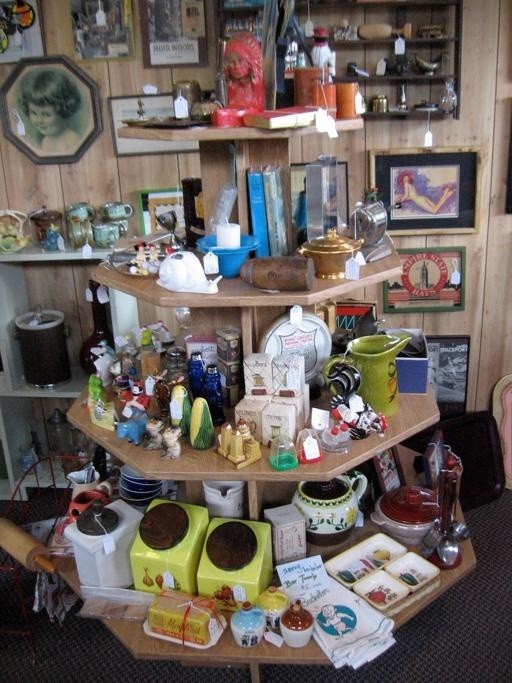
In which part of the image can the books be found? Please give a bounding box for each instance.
[243,106,320,131]
[248,161,338,256]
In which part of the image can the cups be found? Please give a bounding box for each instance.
[108,220,128,237]
[99,201,132,221]
[91,222,119,248]
[30,206,64,250]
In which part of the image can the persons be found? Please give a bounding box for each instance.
[395,170,454,214]
[212,31,263,127]
[19,70,81,153]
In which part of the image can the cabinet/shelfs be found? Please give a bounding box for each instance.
[1,235,180,502]
[55,118,477,683]
[218,0,463,119]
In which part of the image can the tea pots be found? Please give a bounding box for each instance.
[291,475,367,547]
[66,469,112,500]
[323,334,412,417]
[64,202,95,250]
[190,99,223,122]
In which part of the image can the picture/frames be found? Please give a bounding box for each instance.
[69,0,136,64]
[2,55,103,165]
[367,145,482,237]
[426,333,469,418]
[0,0,47,66]
[383,247,466,314]
[137,187,183,238]
[138,0,210,69]
[107,92,199,157]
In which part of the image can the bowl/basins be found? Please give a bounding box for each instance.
[230,602,267,649]
[280,600,314,647]
[260,586,289,628]
[369,486,440,544]
[297,230,366,279]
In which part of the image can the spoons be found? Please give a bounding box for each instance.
[437,482,459,566]
[453,468,470,538]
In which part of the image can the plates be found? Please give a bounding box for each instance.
[119,464,162,504]
[142,613,227,650]
[116,263,158,280]
